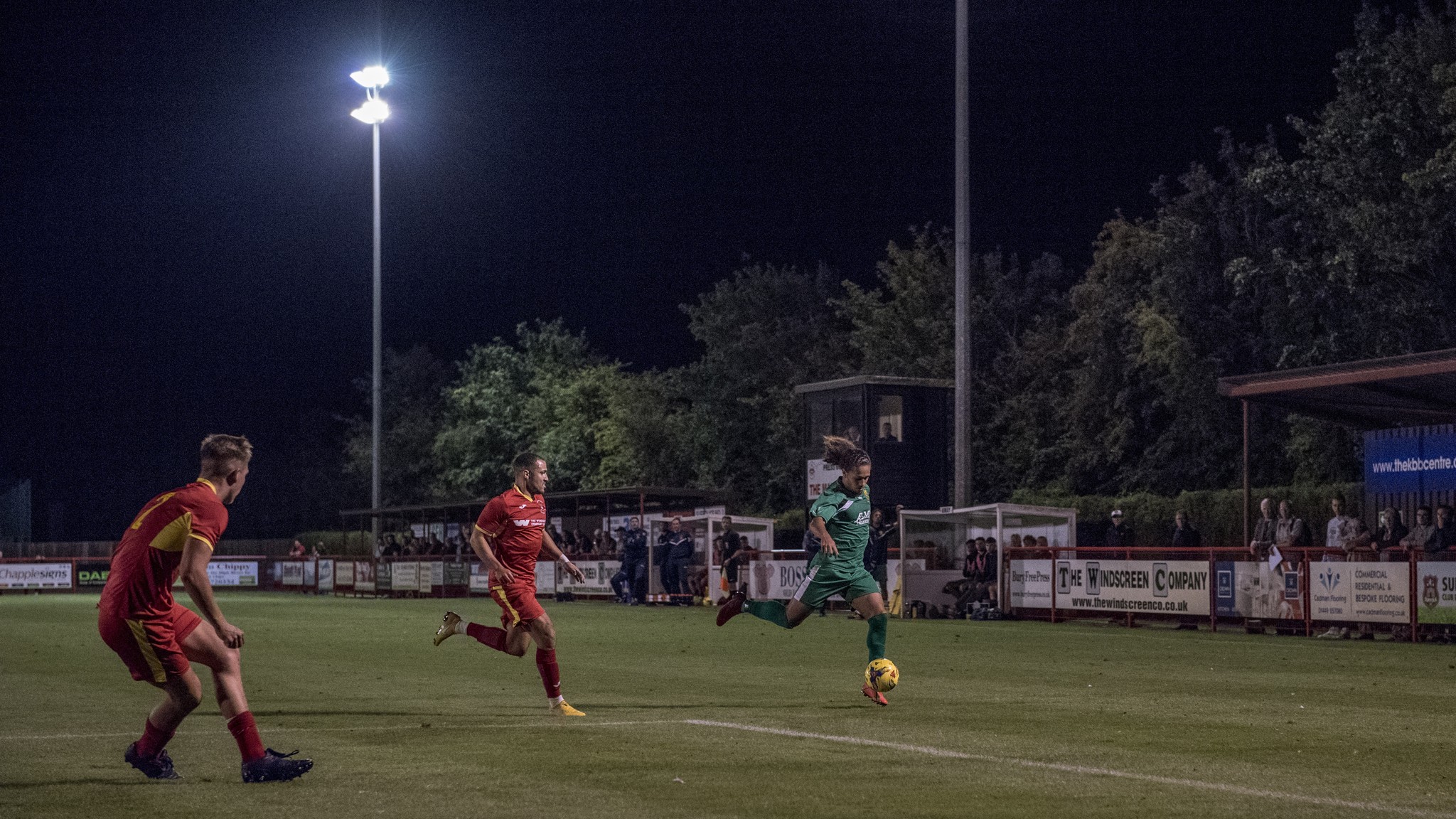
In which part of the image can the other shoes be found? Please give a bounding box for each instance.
[1274,627,1456,641]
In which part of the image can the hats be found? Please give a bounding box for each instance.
[1111,510,1122,517]
[614,526,626,532]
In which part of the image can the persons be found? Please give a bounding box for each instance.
[371,516,757,607]
[95,432,313,784]
[803,526,827,618]
[1317,495,1456,644]
[852,509,898,619]
[433,452,589,717]
[308,541,331,595]
[716,433,892,708]
[946,534,1052,621]
[1093,510,1136,628]
[1163,511,1202,630]
[290,539,307,593]
[904,540,947,619]
[1247,498,1316,637]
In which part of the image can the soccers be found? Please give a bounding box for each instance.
[865,658,899,692]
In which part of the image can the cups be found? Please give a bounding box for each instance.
[966,614,971,621]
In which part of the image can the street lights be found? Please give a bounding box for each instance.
[348,64,391,564]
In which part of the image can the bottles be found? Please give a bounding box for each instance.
[912,605,917,619]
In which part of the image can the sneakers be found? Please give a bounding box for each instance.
[716,591,747,627]
[123,740,185,779]
[549,700,586,716]
[861,682,890,708]
[240,748,315,783]
[433,611,461,646]
